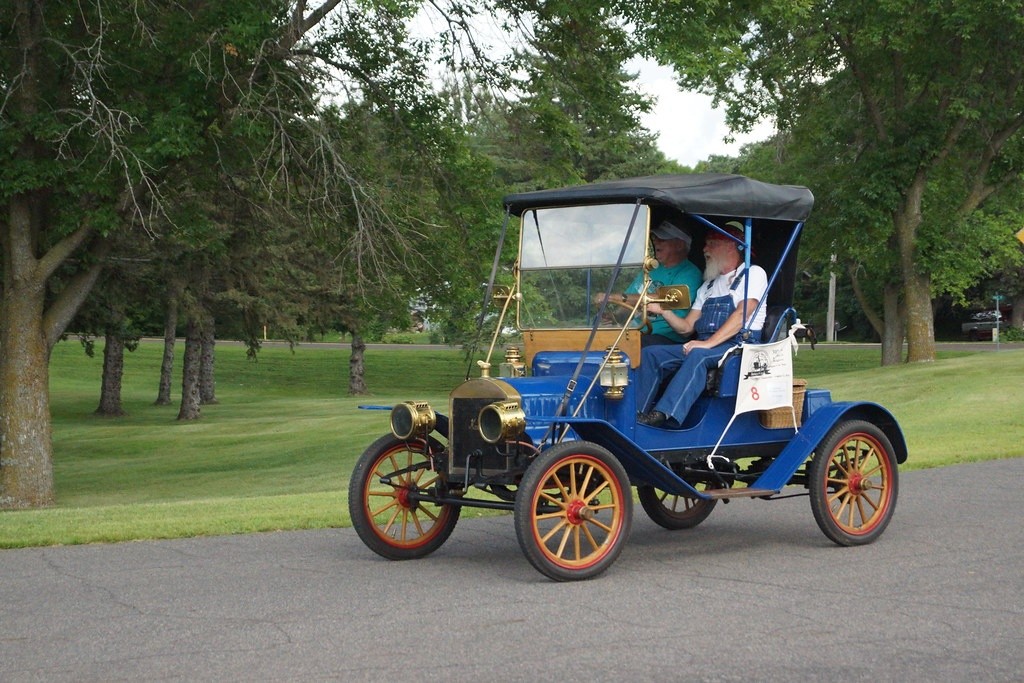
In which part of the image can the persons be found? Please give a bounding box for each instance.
[596,218,702,424]
[634,225,769,430]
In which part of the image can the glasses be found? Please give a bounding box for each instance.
[651,235,658,240]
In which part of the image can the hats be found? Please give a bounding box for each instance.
[651,221,692,244]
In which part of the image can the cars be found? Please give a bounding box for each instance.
[961,309,1006,341]
[347,174,907,581]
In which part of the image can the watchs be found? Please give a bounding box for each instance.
[622,294,627,303]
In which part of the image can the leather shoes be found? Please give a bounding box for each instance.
[637,409,666,427]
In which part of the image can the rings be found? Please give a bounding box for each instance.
[599,299,600,301]
